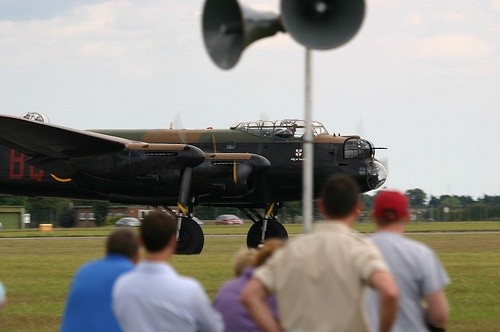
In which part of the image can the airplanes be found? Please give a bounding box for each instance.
[0,115,388,255]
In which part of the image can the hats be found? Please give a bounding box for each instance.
[373,190,409,219]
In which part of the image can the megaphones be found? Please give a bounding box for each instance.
[202,0,280,70]
[280,0,366,50]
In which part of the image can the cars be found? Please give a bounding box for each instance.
[216,214,243,225]
[116,217,141,226]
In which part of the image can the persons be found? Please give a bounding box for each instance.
[58,228,141,332]
[111,210,224,332]
[240,176,399,332]
[214,239,284,332]
[366,190,452,332]
